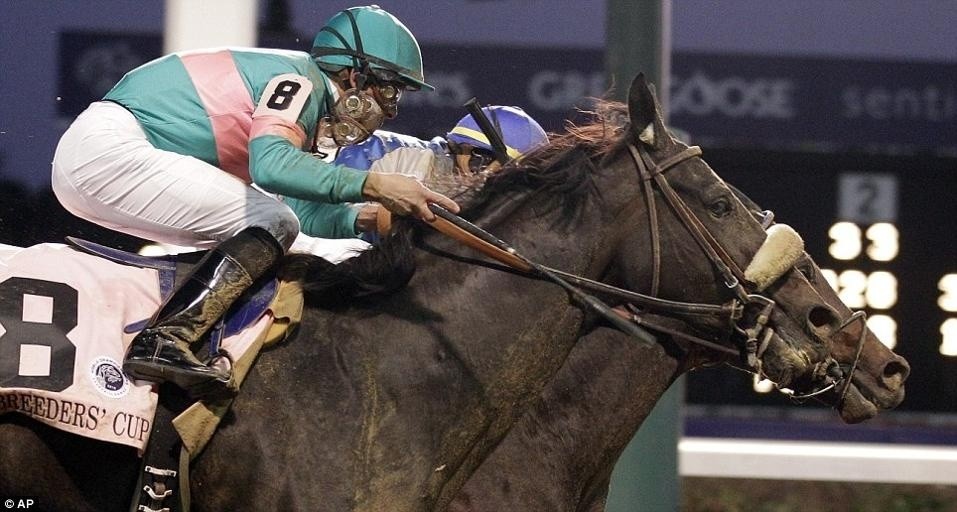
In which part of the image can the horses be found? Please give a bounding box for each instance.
[1,65,844,512]
[443,180,912,512]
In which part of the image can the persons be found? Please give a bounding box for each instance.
[242,102,552,264]
[52,4,462,397]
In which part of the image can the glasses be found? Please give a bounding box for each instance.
[365,66,402,106]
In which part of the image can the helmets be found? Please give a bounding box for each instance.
[448,106,552,159]
[310,4,436,92]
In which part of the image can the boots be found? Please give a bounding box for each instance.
[120,227,286,398]
